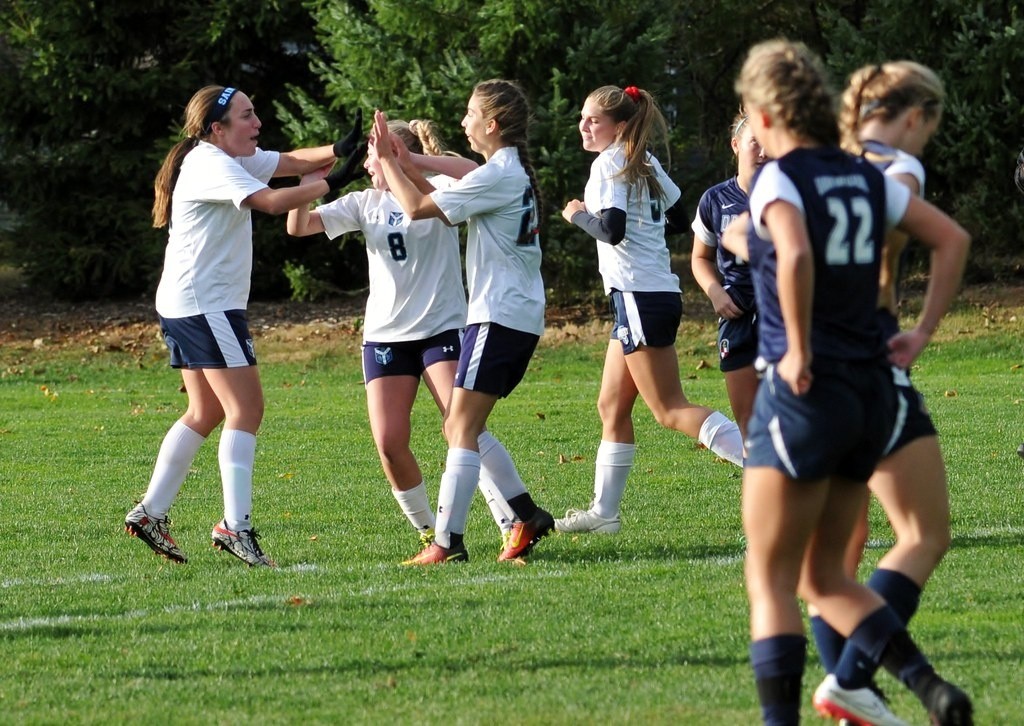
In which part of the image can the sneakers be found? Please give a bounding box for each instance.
[124,502,188,565]
[812,674,910,726]
[399,531,469,565]
[553,506,624,536]
[496,506,554,563]
[211,518,276,569]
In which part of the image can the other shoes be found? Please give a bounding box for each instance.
[417,526,434,548]
[499,531,511,550]
[930,683,974,726]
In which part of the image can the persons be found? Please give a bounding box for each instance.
[123,84,368,568]
[556,86,743,533]
[806,61,952,726]
[286,119,525,566]
[741,35,974,726]
[691,113,767,439]
[370,79,556,565]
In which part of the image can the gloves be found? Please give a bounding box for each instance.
[323,139,368,192]
[333,107,362,158]
